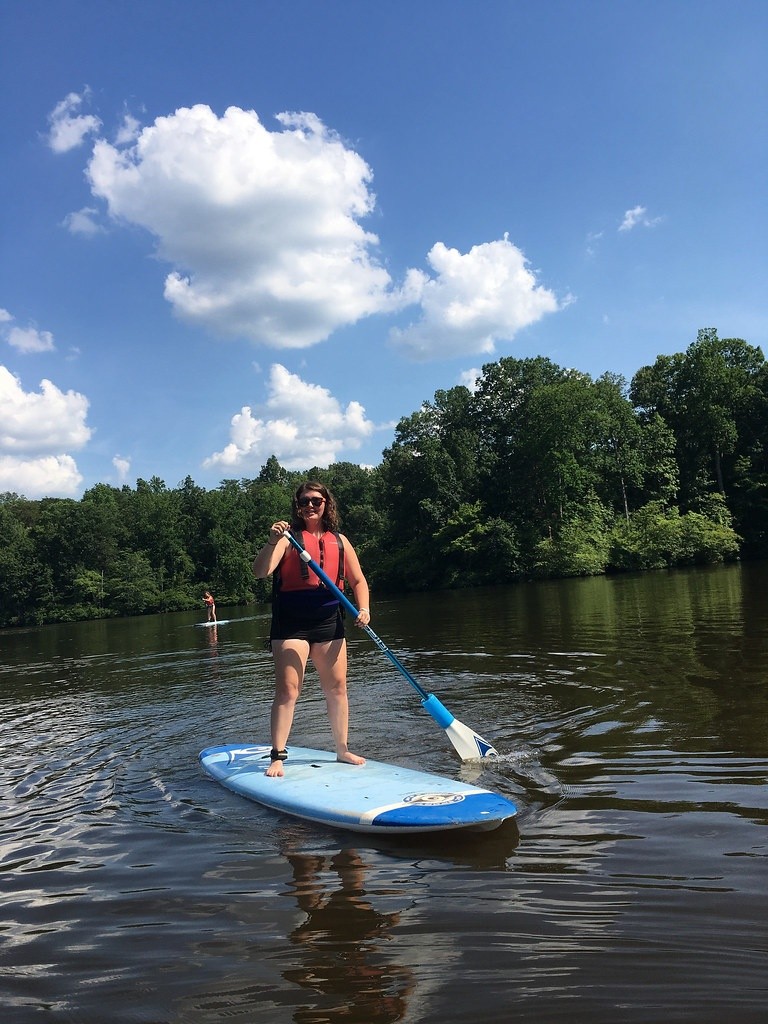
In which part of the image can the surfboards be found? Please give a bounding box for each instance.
[196,741,519,835]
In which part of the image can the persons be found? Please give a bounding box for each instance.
[202,591,218,623]
[207,625,219,649]
[252,482,372,777]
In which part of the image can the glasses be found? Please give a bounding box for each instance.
[297,497,327,508]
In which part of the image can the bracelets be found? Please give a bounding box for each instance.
[359,608,369,612]
[268,541,278,546]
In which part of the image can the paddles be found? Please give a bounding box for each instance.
[281,529,500,760]
